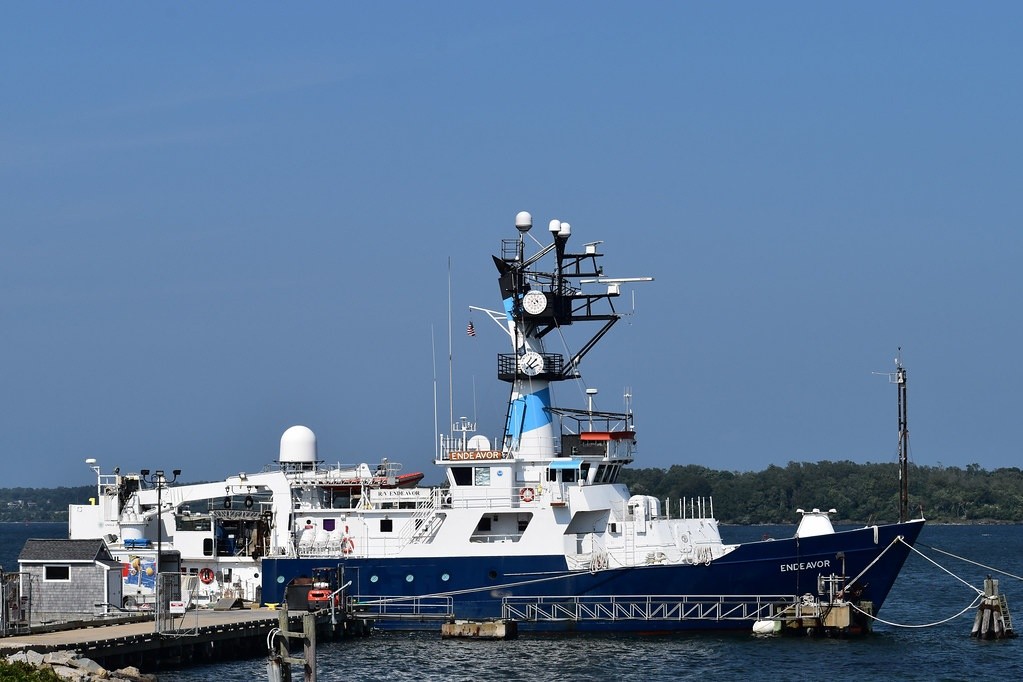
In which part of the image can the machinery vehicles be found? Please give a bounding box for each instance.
[307,566,345,612]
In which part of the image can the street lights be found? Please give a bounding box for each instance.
[141,469,182,573]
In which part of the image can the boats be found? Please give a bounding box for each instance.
[67,211,928,642]
[343,472,425,493]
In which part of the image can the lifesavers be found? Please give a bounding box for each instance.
[342,538,354,554]
[521,487,535,502]
[200,568,214,583]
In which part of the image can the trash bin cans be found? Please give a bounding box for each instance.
[284,577,312,610]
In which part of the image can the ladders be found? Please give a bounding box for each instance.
[273,610,317,682]
[997,594,1013,632]
